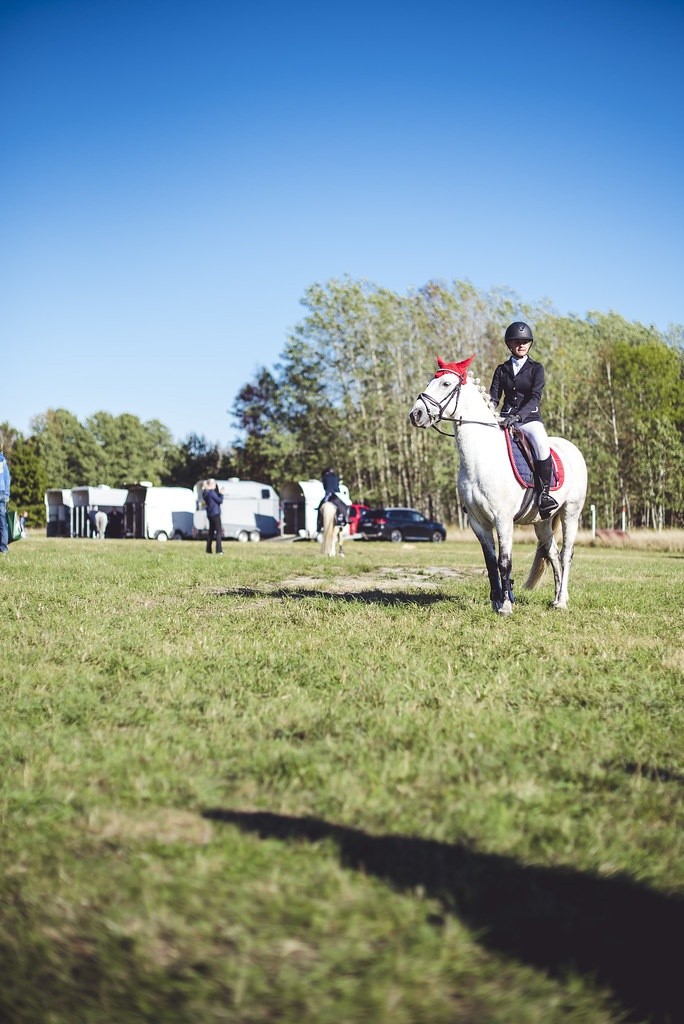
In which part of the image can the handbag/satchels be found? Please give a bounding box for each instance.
[6,501,22,544]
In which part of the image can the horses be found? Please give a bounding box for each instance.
[319,501,345,557]
[409,352,587,618]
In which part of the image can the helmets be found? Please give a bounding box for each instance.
[504,321,533,340]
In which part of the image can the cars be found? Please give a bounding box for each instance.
[348,504,371,536]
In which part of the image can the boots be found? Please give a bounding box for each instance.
[534,454,558,511]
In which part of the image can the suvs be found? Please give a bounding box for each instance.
[356,507,447,544]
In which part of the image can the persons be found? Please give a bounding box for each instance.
[202,478,225,556]
[317,465,354,523]
[19,511,28,535]
[0,434,11,553]
[460,322,559,515]
[88,505,124,539]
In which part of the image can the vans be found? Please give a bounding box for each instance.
[192,477,286,543]
[124,482,194,543]
